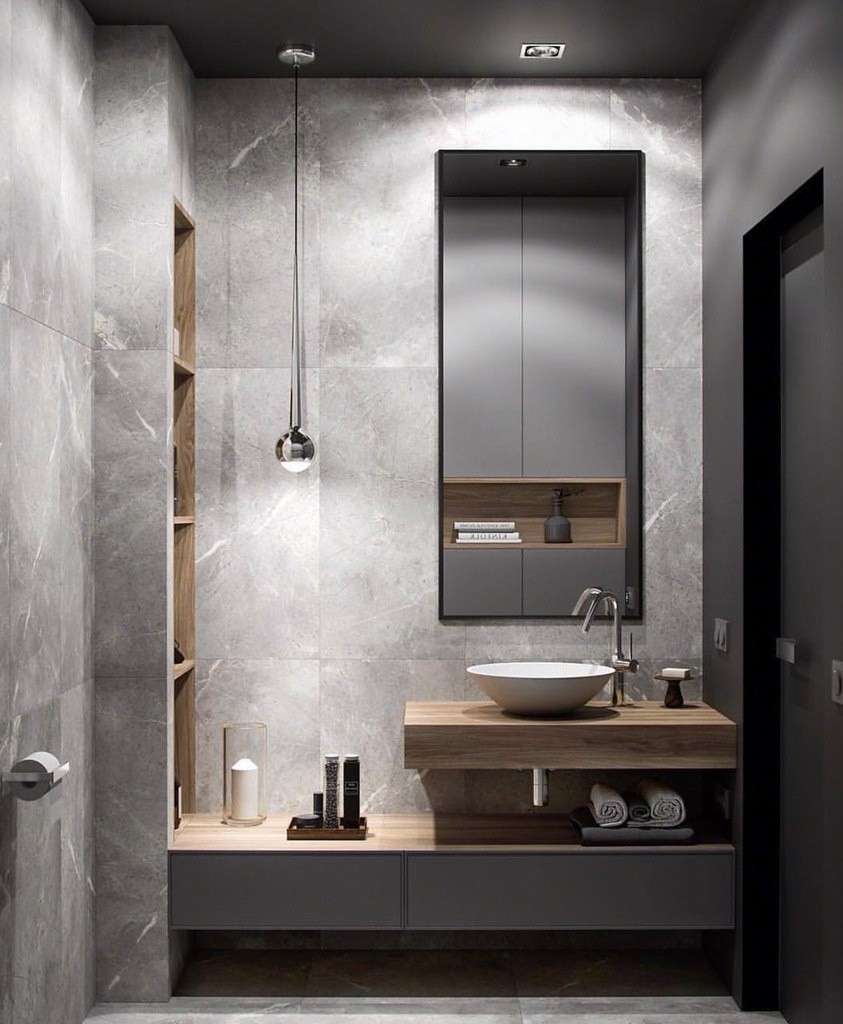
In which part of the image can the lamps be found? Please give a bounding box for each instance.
[276,42,318,474]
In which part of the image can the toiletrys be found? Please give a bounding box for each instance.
[296,814,320,829]
[313,792,324,830]
[342,754,361,830]
[323,754,341,829]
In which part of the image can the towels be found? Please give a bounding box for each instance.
[558,777,695,846]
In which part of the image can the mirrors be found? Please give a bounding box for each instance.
[437,149,647,621]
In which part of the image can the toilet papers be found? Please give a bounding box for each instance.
[10,751,63,800]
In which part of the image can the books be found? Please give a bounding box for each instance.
[453,521,523,544]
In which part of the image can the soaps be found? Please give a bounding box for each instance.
[661,667,691,680]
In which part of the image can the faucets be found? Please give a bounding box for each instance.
[581,590,625,706]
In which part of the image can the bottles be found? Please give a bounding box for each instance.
[222,721,267,827]
[342,752,360,829]
[321,754,341,829]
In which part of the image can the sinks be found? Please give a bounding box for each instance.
[464,661,618,720]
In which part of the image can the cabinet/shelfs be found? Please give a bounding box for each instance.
[170,196,199,839]
